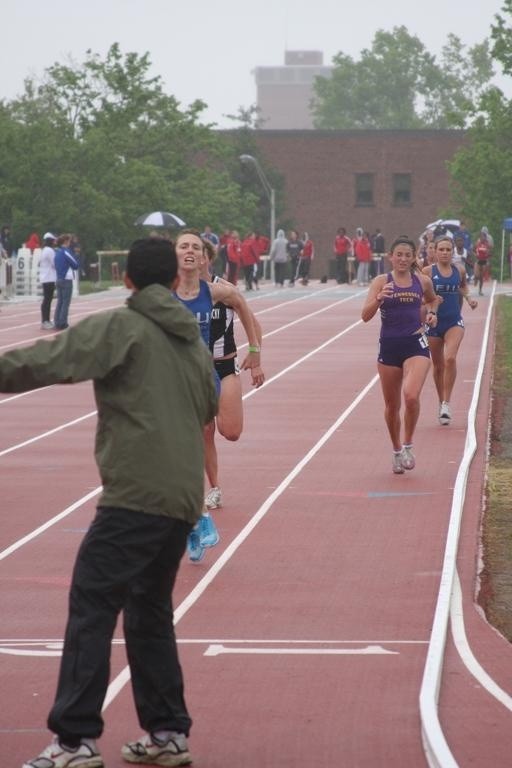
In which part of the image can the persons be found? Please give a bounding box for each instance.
[199,238,265,510]
[421,238,478,424]
[361,237,438,477]
[0,240,219,768]
[0,227,82,331]
[171,228,260,561]
[201,222,494,297]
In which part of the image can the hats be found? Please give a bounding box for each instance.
[43,232,58,240]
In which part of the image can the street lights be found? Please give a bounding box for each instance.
[238,154,276,285]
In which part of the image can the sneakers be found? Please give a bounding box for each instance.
[22,737,102,768]
[393,449,414,475]
[438,399,450,426]
[187,488,222,563]
[120,731,193,768]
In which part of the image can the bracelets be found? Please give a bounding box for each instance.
[248,346,261,352]
[375,297,382,302]
[429,312,436,315]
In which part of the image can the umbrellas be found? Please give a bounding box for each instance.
[133,210,187,228]
[425,218,462,238]
